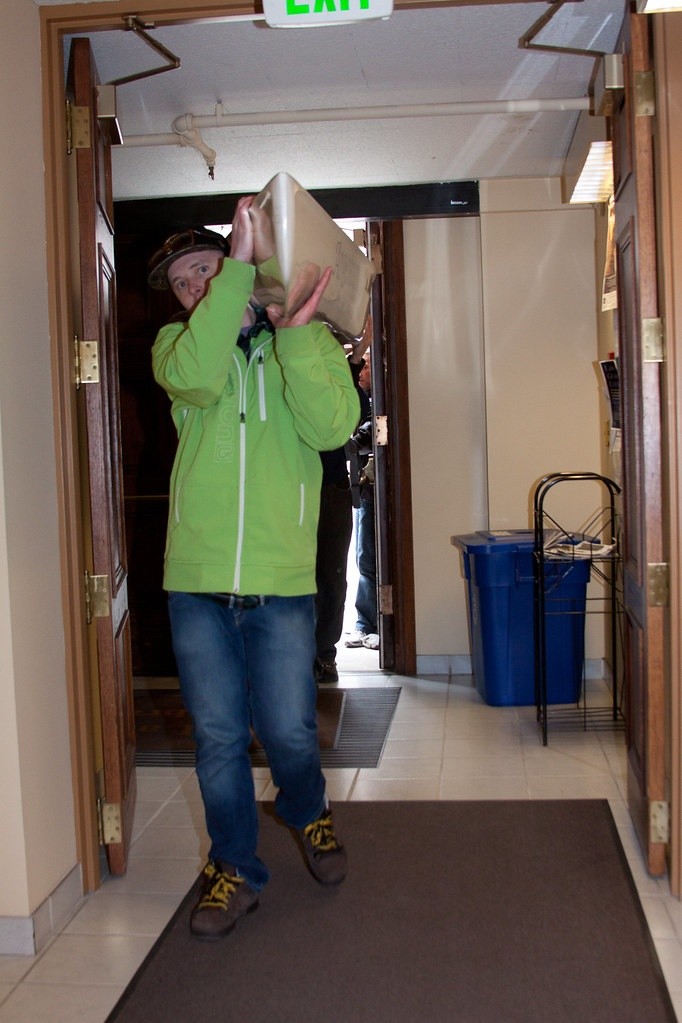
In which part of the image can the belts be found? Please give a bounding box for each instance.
[187,593,274,609]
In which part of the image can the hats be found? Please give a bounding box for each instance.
[147,222,227,291]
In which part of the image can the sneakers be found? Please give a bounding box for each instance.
[297,806,349,886]
[189,860,257,933]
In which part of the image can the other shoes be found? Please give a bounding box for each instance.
[316,656,339,687]
[344,630,362,649]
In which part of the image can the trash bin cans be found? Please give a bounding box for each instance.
[448,528,602,708]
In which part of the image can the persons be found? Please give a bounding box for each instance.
[152,195,361,940]
[314,317,379,683]
